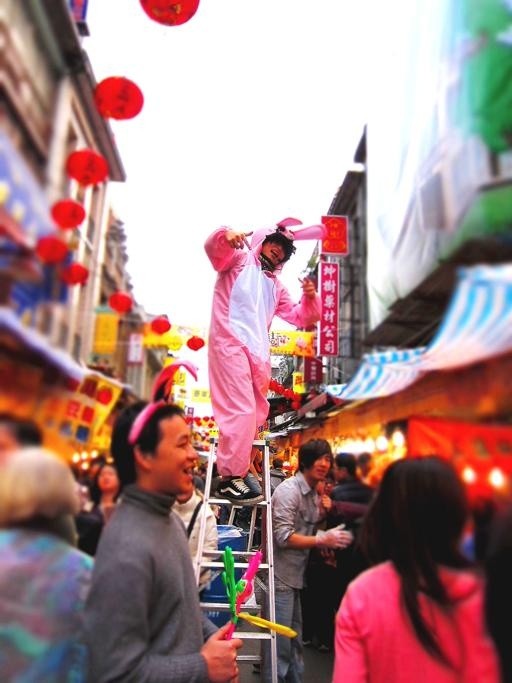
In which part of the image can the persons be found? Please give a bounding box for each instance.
[85,402,244,683]
[173,458,263,585]
[271,438,512,683]
[0,408,119,683]
[204,216,328,507]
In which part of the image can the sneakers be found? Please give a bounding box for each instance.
[214,475,266,506]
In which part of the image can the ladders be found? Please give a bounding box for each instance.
[193,438,278,682]
